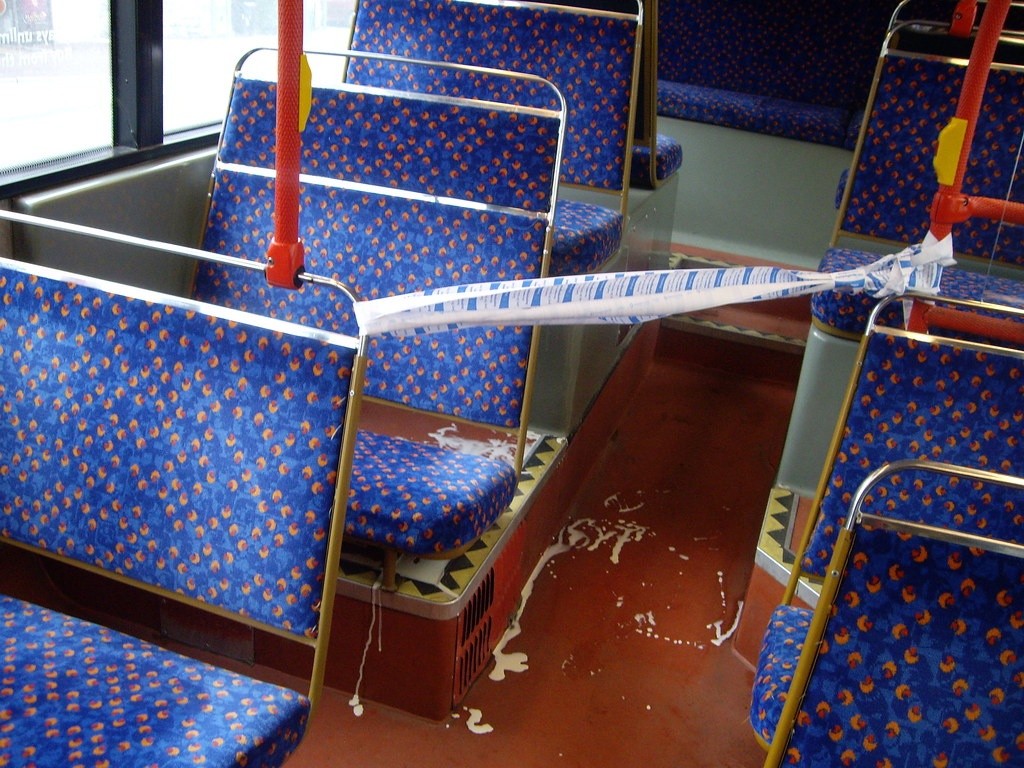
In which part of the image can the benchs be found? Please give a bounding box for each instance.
[508,0,684,192]
[751,293,1024,752]
[763,458,1024,768]
[1,210,368,768]
[343,0,643,278]
[835,0,1024,210]
[812,21,1024,352]
[657,0,1024,152]
[187,46,568,587]
